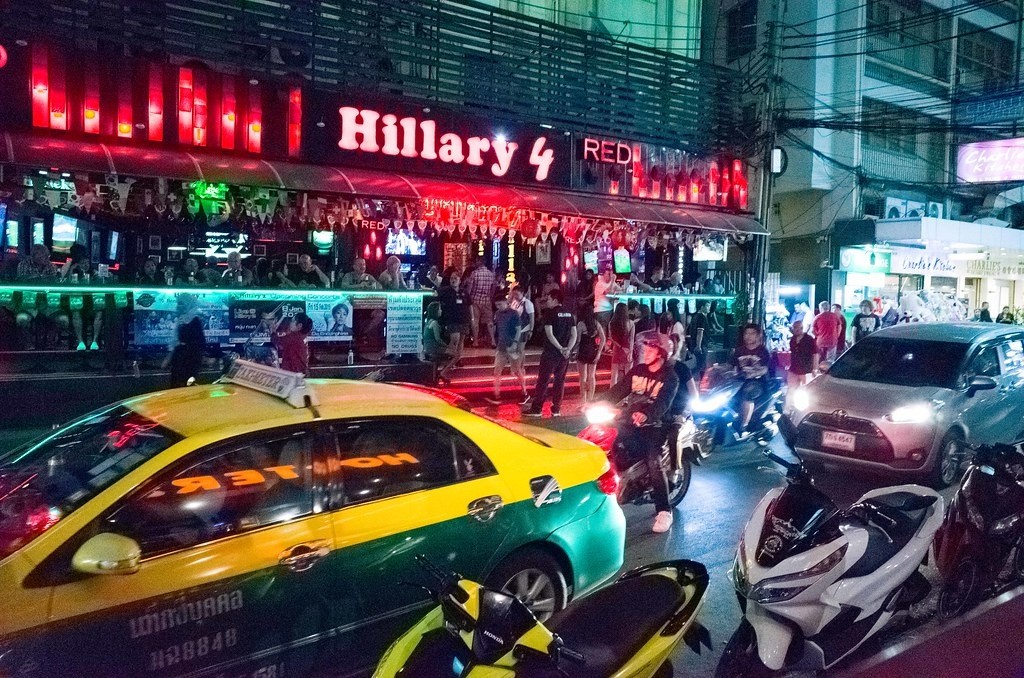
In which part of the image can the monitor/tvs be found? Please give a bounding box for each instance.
[386,228,429,256]
[584,248,632,274]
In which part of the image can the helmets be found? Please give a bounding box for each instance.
[642,333,674,361]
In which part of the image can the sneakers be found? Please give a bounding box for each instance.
[550,410,561,416]
[521,408,542,417]
[652,510,673,532]
[668,469,679,484]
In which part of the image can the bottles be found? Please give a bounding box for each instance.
[167,268,173,287]
[189,260,195,276]
[228,272,234,290]
[504,346,520,361]
[347,350,354,365]
[364,275,369,289]
[132,360,142,379]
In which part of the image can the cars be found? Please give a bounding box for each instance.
[0,359,626,678]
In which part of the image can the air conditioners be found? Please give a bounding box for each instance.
[906,200,925,217]
[92,0,340,84]
[926,201,944,218]
[875,197,906,219]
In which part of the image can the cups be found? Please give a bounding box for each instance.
[84,273,90,284]
[189,277,194,281]
[638,290,643,295]
[407,280,415,291]
[70,274,78,283]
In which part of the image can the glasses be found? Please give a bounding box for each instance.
[431,271,436,275]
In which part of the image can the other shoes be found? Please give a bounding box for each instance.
[738,428,748,439]
[517,393,530,404]
[77,342,86,350]
[575,405,583,412]
[90,342,99,350]
[484,397,502,404]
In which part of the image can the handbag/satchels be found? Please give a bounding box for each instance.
[622,347,632,362]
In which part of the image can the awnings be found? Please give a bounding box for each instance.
[0,125,772,244]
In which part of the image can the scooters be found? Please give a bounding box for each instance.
[688,364,785,457]
[366,554,710,678]
[715,443,1024,678]
[575,402,706,510]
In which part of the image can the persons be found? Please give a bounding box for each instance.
[15,245,1018,534]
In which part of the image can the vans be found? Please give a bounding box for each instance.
[781,322,1024,489]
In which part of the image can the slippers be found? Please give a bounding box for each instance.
[437,375,451,383]
[491,344,497,350]
[470,344,479,348]
